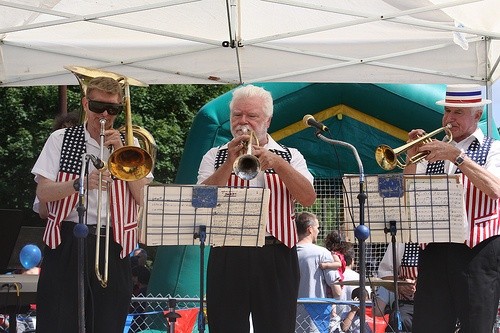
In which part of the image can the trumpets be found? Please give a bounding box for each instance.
[375,123,454,170]
[233,126,261,182]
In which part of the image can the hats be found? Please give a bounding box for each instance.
[435,83,492,106]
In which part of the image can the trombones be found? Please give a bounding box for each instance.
[95,75,155,287]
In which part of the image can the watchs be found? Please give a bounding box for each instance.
[453,151,466,167]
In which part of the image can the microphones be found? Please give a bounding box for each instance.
[89,154,108,172]
[303,114,329,132]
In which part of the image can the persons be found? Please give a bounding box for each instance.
[196,84,317,333]
[320,230,416,333]
[31,77,154,333]
[296,212,343,333]
[403,83,500,333]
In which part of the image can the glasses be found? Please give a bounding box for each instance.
[88,96,123,115]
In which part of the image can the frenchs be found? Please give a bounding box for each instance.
[62,64,157,174]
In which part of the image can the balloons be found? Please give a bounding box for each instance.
[19,244,42,270]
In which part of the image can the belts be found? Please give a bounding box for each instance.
[265,238,282,244]
[61,223,113,234]
